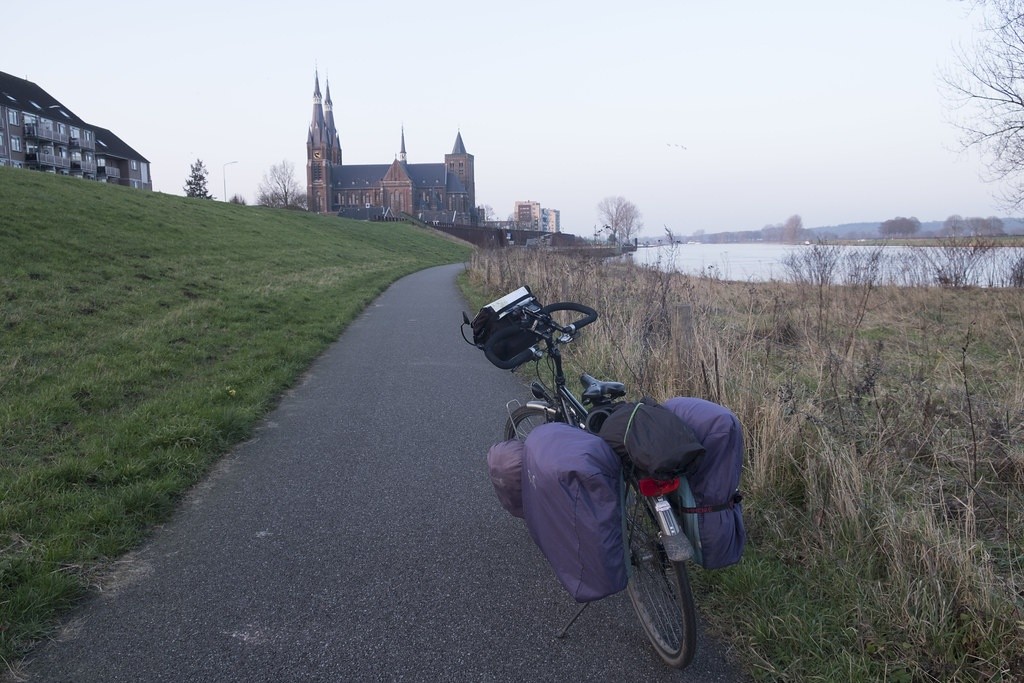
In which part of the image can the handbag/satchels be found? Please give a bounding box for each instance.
[470,285,547,361]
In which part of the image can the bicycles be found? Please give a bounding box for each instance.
[483,301,698,669]
